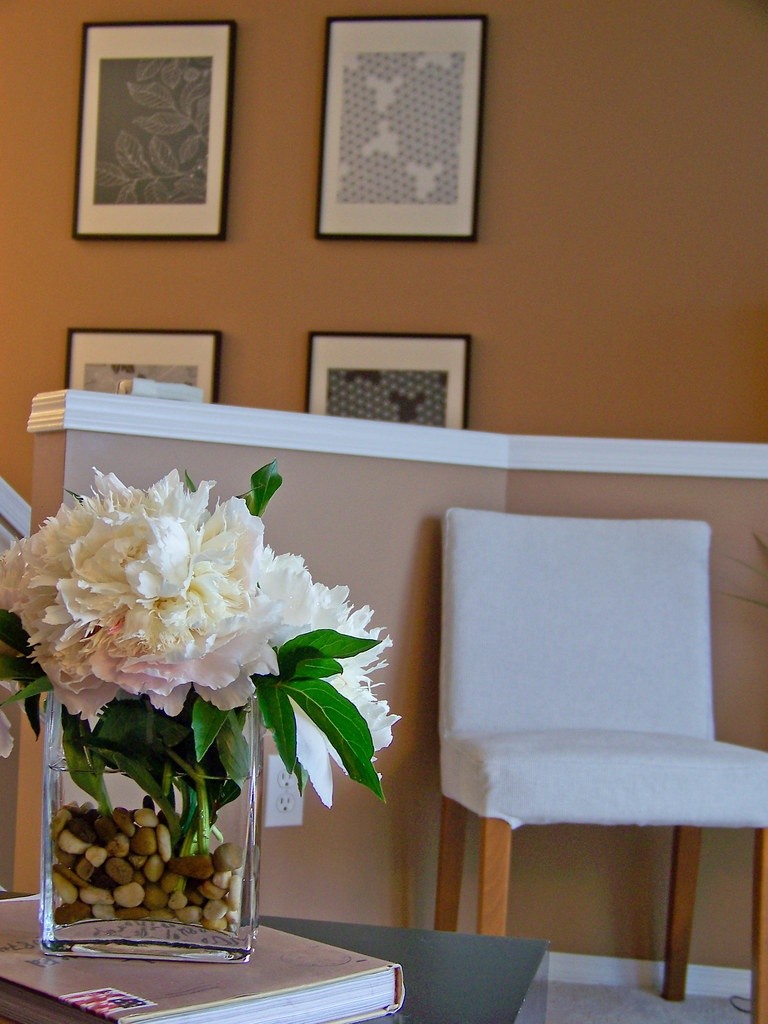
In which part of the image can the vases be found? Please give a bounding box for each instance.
[39,681,265,965]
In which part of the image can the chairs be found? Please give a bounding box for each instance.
[431,511,768,1024]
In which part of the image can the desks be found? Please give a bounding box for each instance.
[0,891,550,1024]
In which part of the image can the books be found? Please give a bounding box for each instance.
[0,892,406,1024]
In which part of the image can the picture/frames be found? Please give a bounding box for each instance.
[303,330,472,429]
[63,326,221,403]
[69,21,238,240]
[312,15,486,240]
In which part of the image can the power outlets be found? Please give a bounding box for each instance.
[262,752,303,827]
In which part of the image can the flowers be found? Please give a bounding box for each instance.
[0,457,402,897]
